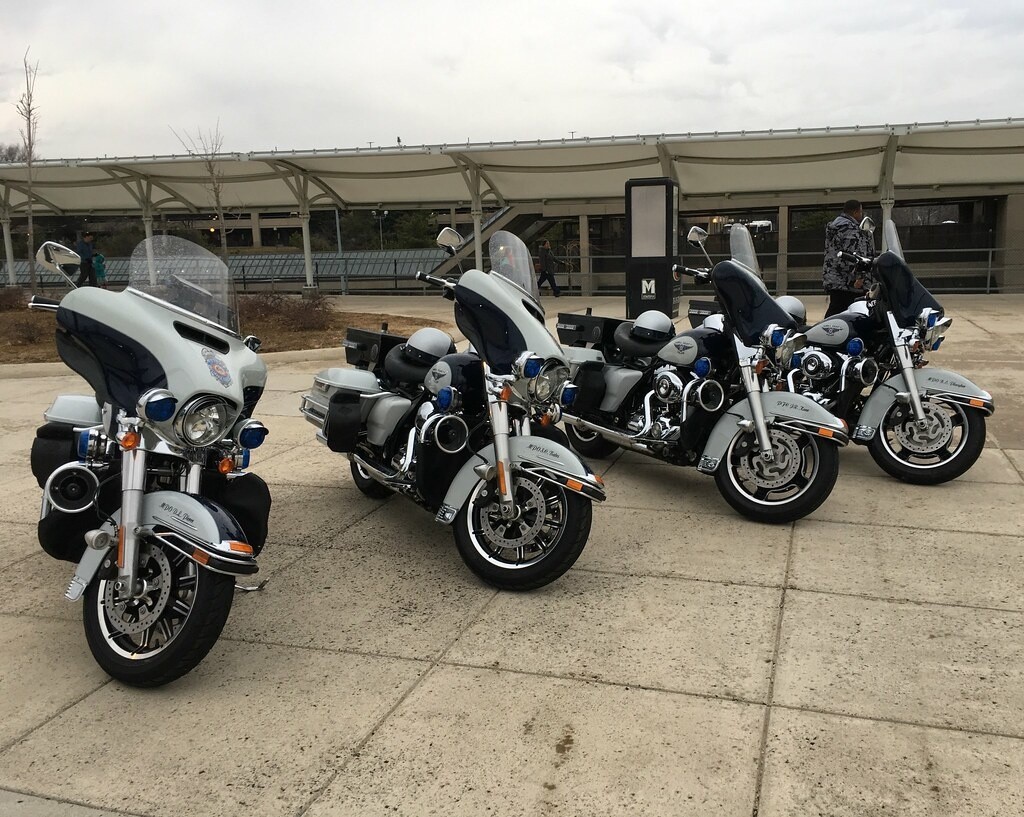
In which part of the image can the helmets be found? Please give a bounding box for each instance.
[775,296,806,325]
[629,310,676,341]
[403,328,456,367]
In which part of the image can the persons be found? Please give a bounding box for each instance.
[821,199,875,318]
[74,233,100,286]
[92,254,107,288]
[538,240,564,297]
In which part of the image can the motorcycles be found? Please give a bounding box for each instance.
[298,226,607,591]
[26,234,273,682]
[543,222,852,524]
[687,217,996,487]
